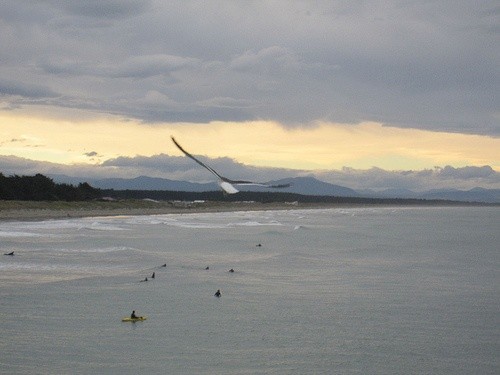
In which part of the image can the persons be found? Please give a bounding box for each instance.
[131,310,138,319]
[215,289,221,297]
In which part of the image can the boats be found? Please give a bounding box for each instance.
[122,316,148,321]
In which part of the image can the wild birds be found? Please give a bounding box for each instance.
[172,137,290,194]
